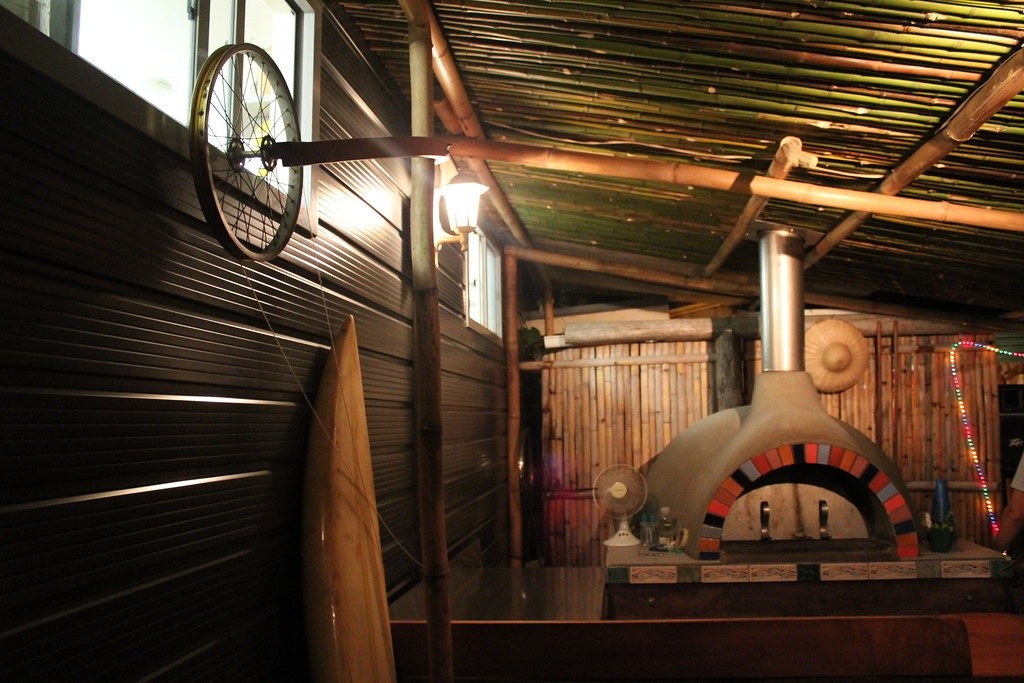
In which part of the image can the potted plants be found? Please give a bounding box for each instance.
[924,508,960,553]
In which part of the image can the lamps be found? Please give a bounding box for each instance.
[435,159,490,270]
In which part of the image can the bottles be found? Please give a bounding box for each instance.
[640,498,658,547]
[658,507,673,547]
[931,480,949,524]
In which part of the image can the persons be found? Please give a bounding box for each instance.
[991,448,1024,551]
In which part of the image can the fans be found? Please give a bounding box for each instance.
[591,463,648,547]
